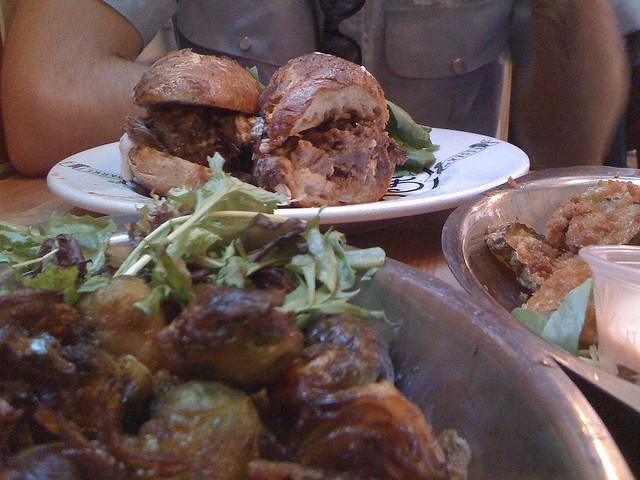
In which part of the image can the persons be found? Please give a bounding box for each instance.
[1,0,630,178]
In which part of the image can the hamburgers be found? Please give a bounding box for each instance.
[121,47,262,196]
[252,52,409,208]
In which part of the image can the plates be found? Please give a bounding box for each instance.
[46,125,530,238]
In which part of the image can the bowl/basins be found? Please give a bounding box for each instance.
[441,164,640,413]
[0,210,634,480]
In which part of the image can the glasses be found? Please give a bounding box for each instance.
[318,0,365,65]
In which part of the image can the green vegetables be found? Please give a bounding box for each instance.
[0,150,387,326]
